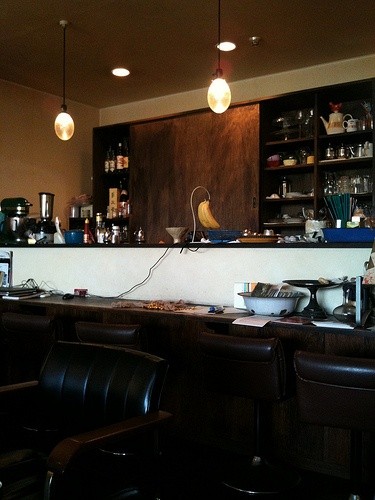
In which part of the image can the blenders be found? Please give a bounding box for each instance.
[34,192,57,243]
[0,197,33,246]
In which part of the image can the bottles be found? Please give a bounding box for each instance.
[83,212,145,244]
[333,288,356,322]
[103,141,129,218]
[365,110,373,130]
[278,176,289,199]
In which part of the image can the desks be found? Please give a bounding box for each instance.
[0,288,375,370]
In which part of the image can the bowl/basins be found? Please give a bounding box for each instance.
[283,157,298,166]
[207,230,242,243]
[238,291,306,316]
[65,230,83,244]
[266,159,281,168]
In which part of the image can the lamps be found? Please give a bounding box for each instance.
[54,20,74,141]
[208,0,231,113]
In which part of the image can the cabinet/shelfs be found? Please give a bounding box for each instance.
[257,76,375,241]
[93,123,136,245]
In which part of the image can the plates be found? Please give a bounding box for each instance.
[235,236,280,244]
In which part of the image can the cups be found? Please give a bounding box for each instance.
[342,119,358,133]
[325,141,373,160]
[307,156,314,164]
[322,175,373,194]
[305,219,326,242]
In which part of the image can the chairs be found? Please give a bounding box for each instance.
[0,310,375,500]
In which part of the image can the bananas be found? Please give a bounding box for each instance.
[198,199,220,229]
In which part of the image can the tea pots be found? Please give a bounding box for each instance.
[319,109,353,135]
